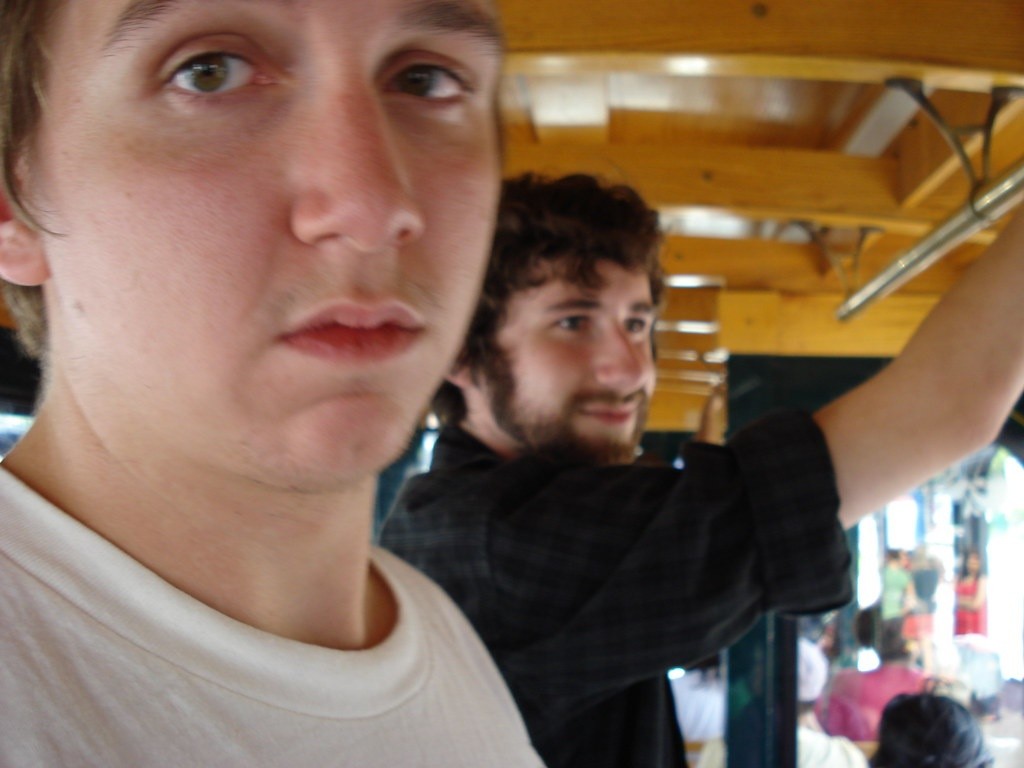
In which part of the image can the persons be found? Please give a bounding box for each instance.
[669,550,1005,768]
[1,0,546,768]
[376,170,1024,768]
